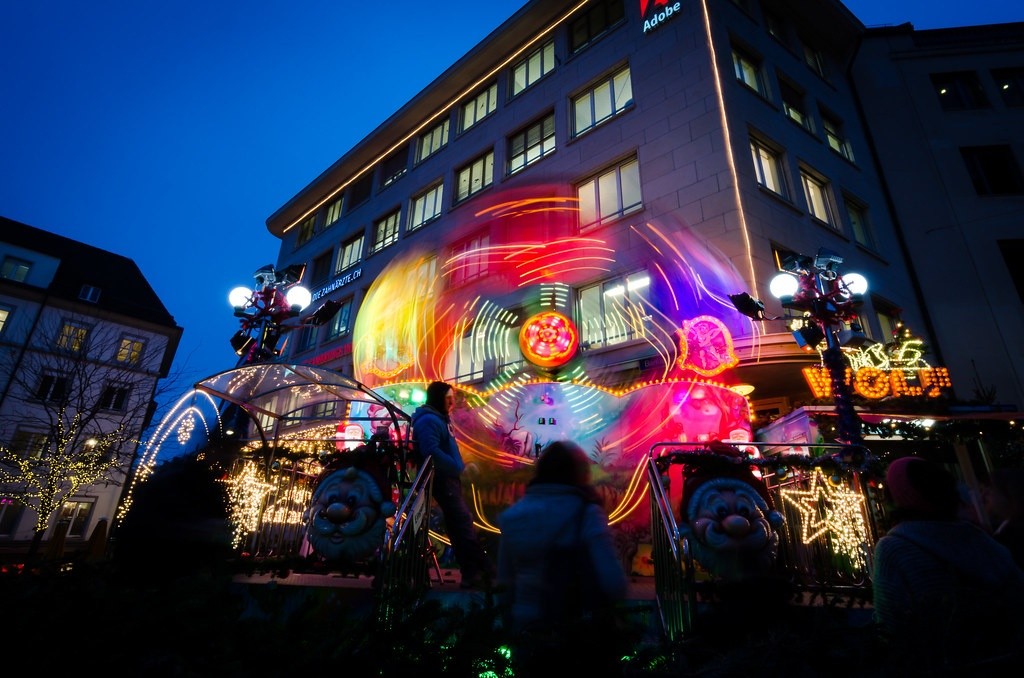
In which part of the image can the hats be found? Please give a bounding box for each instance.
[535,440,596,469]
[887,457,932,507]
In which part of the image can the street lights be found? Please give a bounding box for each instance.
[763,262,881,544]
[220,263,314,453]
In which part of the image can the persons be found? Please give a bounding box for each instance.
[500,440,628,678]
[410,381,500,591]
[872,457,1016,678]
